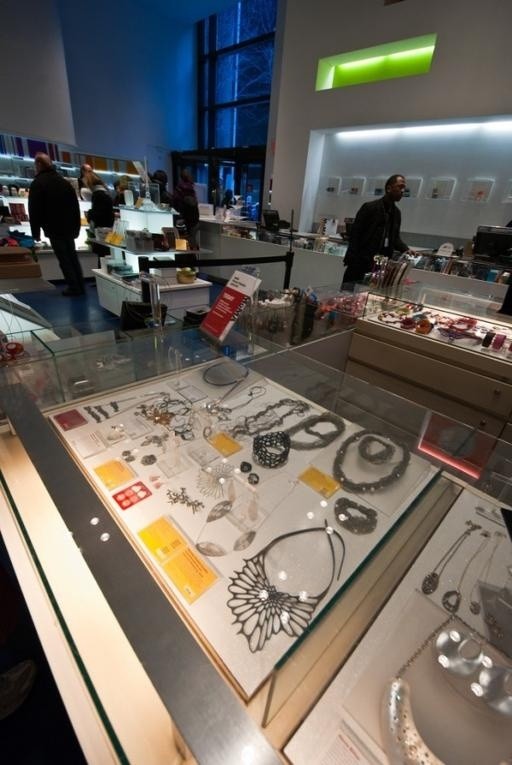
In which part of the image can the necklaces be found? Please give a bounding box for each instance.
[331,429,410,494]
[193,478,301,559]
[279,411,346,451]
[227,396,311,438]
[377,607,511,765]
[208,379,271,413]
[421,519,511,644]
[84,391,166,424]
[223,520,348,655]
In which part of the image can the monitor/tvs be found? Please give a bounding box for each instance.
[264,210,280,233]
[474,226,512,268]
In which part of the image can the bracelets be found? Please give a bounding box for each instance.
[490,334,506,350]
[400,316,416,330]
[333,498,378,535]
[251,431,292,467]
[413,319,433,334]
[481,332,498,348]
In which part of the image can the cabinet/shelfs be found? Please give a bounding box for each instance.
[80,169,152,279]
[0,154,141,282]
[86,206,214,317]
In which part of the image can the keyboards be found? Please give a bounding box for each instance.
[472,259,509,268]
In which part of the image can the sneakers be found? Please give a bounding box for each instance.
[63,288,84,295]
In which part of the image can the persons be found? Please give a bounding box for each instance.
[85,172,115,288]
[27,152,86,297]
[220,189,237,210]
[75,163,93,197]
[338,173,421,292]
[239,183,259,221]
[118,174,140,206]
[111,179,127,207]
[164,167,200,249]
[150,169,172,204]
